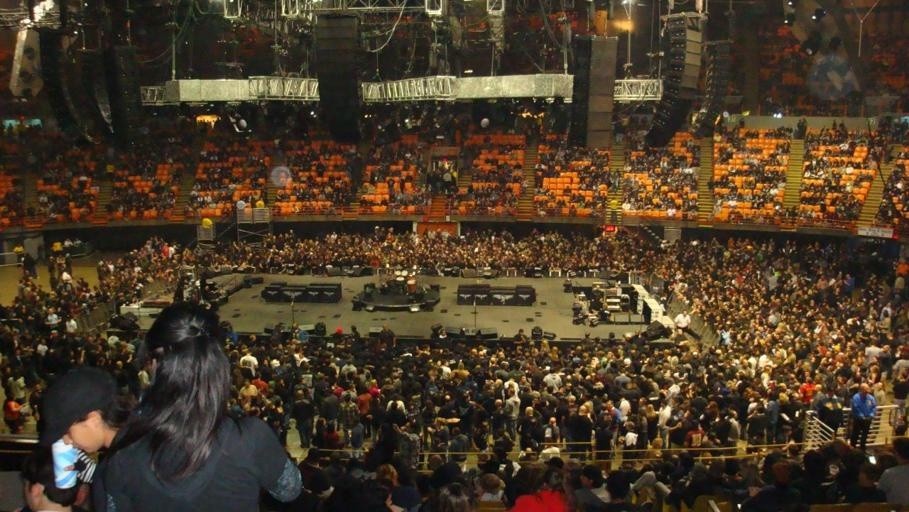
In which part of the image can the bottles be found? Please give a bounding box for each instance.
[51,434,81,490]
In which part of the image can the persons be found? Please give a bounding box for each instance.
[2,108,909,511]
[730,25,908,106]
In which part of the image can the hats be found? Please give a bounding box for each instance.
[36,364,112,447]
[543,457,564,468]
